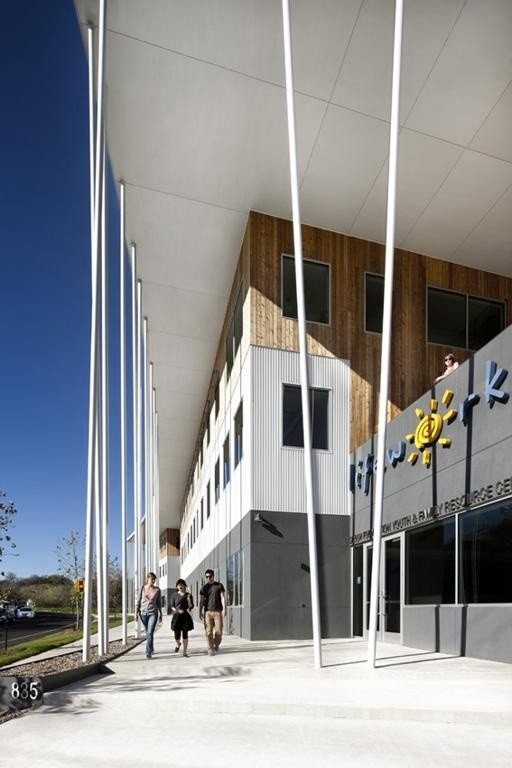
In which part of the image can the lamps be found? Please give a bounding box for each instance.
[254,513,263,524]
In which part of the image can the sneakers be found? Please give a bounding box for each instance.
[145,644,222,660]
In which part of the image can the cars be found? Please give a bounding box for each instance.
[0,604,36,628]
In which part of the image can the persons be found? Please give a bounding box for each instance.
[134,572,164,661]
[434,353,460,383]
[198,568,228,656]
[169,579,196,658]
